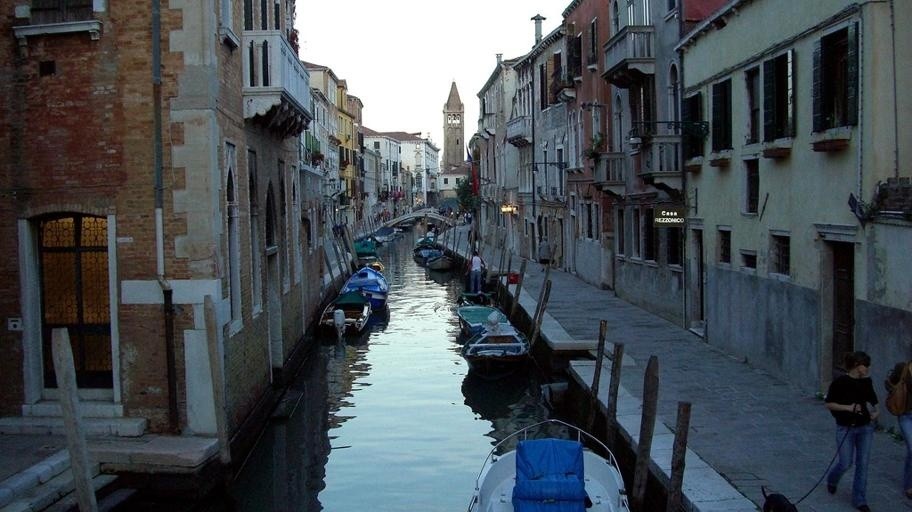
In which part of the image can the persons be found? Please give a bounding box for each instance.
[372,204,400,226]
[467,225,478,251]
[466,250,485,293]
[883,358,912,500]
[823,350,881,512]
[538,236,551,272]
[438,207,472,224]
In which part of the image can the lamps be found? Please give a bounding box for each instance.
[626,117,710,149]
[529,161,568,175]
[500,203,520,221]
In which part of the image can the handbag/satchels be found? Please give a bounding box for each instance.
[884,364,908,417]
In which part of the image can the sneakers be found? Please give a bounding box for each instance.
[858,504,870,511]
[827,473,837,494]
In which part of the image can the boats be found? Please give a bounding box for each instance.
[318,216,405,334]
[413,220,458,280]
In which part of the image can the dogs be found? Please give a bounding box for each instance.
[760,484,798,512]
